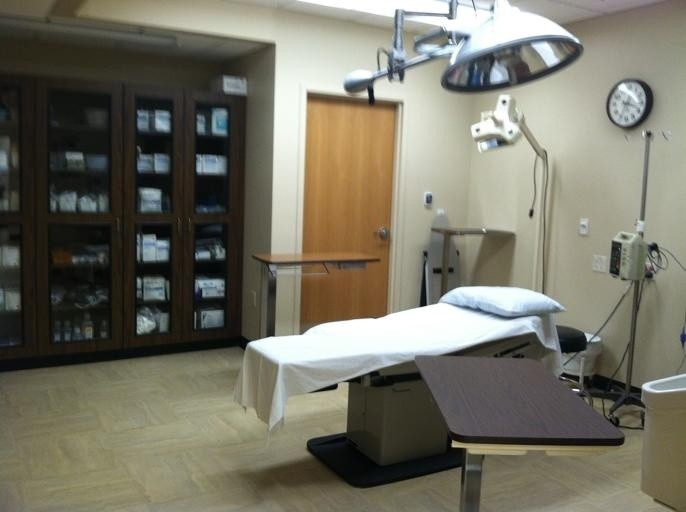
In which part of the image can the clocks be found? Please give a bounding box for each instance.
[606,78,653,129]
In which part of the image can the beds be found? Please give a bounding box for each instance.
[230,287,565,489]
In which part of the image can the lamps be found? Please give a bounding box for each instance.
[0,17,181,52]
[439,1,584,93]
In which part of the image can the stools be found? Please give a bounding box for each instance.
[555,324,594,409]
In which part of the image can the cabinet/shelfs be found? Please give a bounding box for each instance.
[0,67,247,364]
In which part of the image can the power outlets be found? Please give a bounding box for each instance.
[643,262,656,281]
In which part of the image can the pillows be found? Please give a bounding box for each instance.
[436,284,568,320]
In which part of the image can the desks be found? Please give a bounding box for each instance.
[251,252,381,393]
[414,355,626,512]
